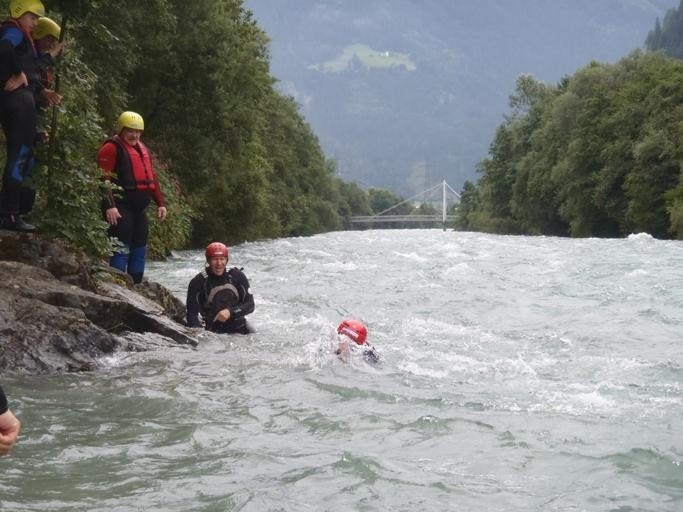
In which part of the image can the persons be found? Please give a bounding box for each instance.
[185,242,255,336]
[0,385,20,454]
[332,319,381,364]
[97,111,167,283]
[0,0,64,232]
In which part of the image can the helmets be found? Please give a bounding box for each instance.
[338,319,367,346]
[115,110,145,136]
[8,0,45,20]
[31,17,61,42]
[205,241,228,259]
